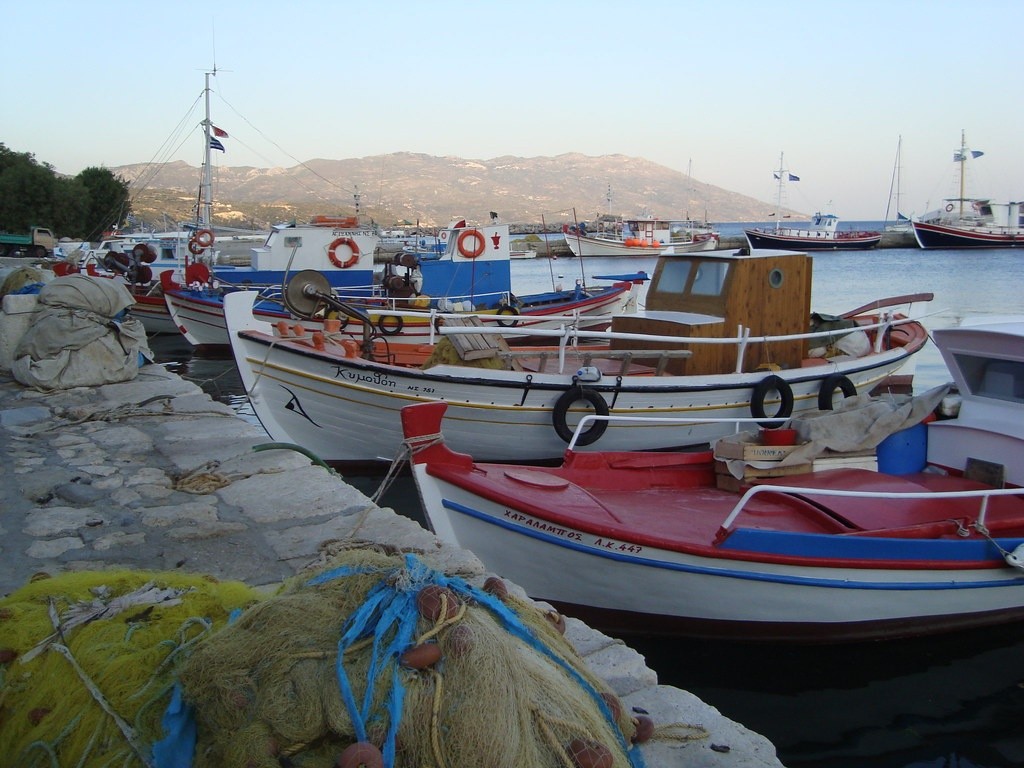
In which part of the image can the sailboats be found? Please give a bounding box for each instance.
[879,135,917,247]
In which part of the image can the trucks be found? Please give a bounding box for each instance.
[0,227,57,258]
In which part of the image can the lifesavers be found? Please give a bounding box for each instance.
[553,388,609,450]
[598,223,604,229]
[818,373,857,411]
[750,374,794,430]
[189,237,204,254]
[616,221,624,229]
[378,315,403,335]
[196,229,215,247]
[496,306,518,327]
[441,232,446,239]
[328,237,359,268]
[458,229,486,258]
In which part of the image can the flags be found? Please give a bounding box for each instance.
[783,216,790,218]
[972,150,984,158]
[204,126,228,152]
[789,174,800,181]
[898,211,908,220]
[774,174,780,179]
[953,153,967,162]
[768,213,775,216]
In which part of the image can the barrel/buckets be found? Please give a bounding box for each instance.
[577,366,603,382]
[758,427,795,446]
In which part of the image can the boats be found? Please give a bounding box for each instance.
[400,322,1024,644]
[58,24,934,475]
[741,152,881,252]
[910,129,1024,250]
[563,215,720,257]
